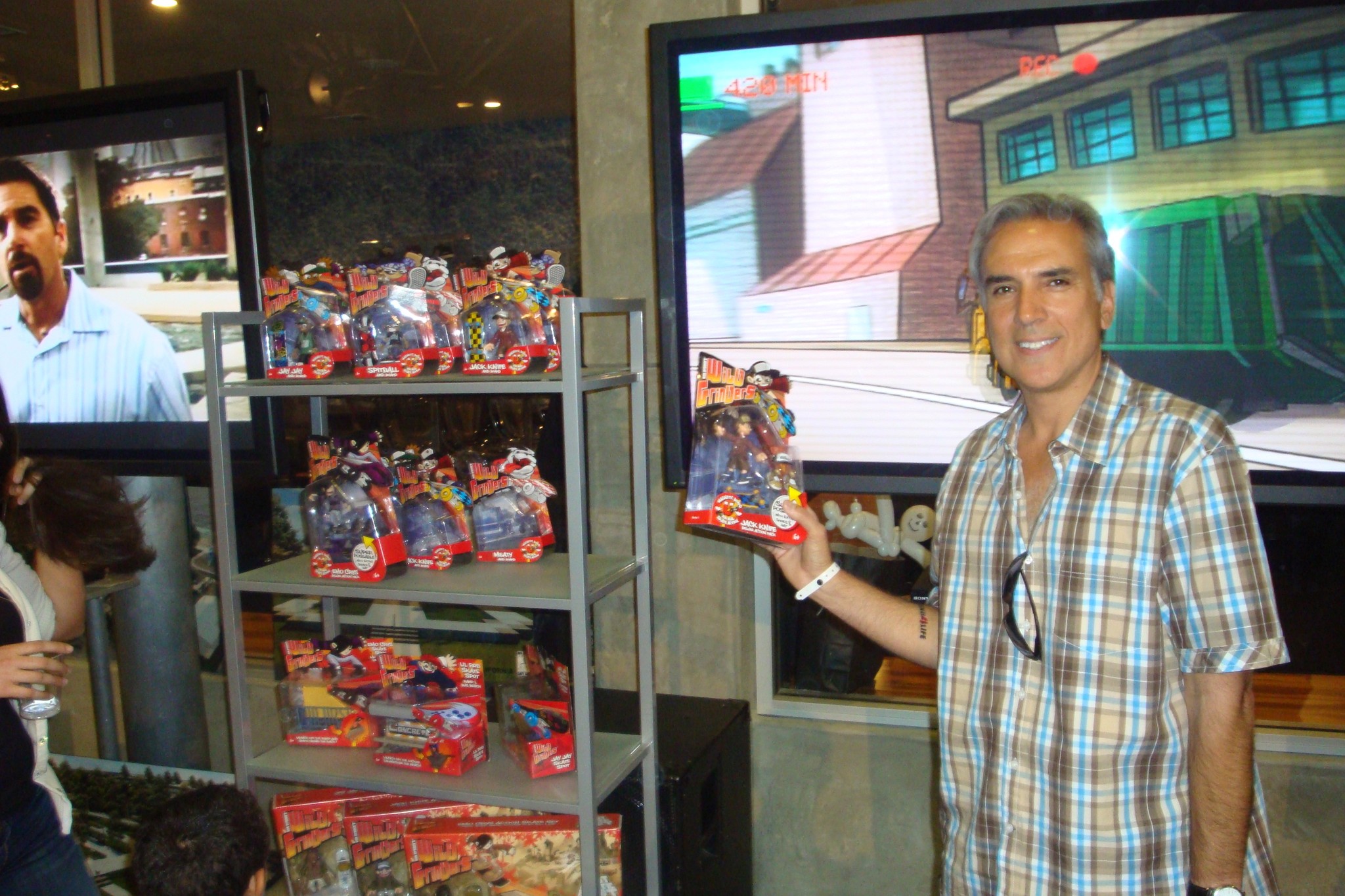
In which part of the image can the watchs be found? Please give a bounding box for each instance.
[1187,880,1245,896]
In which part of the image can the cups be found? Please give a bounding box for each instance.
[17,650,66,721]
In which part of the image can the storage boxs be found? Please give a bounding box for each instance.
[682,351,807,545]
[260,249,623,896]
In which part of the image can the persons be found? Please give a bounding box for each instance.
[760,191,1290,896]
[131,786,271,896]
[0,391,157,896]
[292,310,521,554]
[715,413,768,484]
[0,156,192,423]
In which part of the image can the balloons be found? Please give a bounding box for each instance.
[821,494,936,567]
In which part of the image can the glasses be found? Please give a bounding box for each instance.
[81,568,103,585]
[1002,552,1042,661]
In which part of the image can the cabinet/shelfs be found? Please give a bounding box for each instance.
[201,298,659,896]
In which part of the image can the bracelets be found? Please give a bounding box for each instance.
[796,562,841,600]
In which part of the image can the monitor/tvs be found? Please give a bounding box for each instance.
[0,69,293,475]
[645,0,1345,510]
